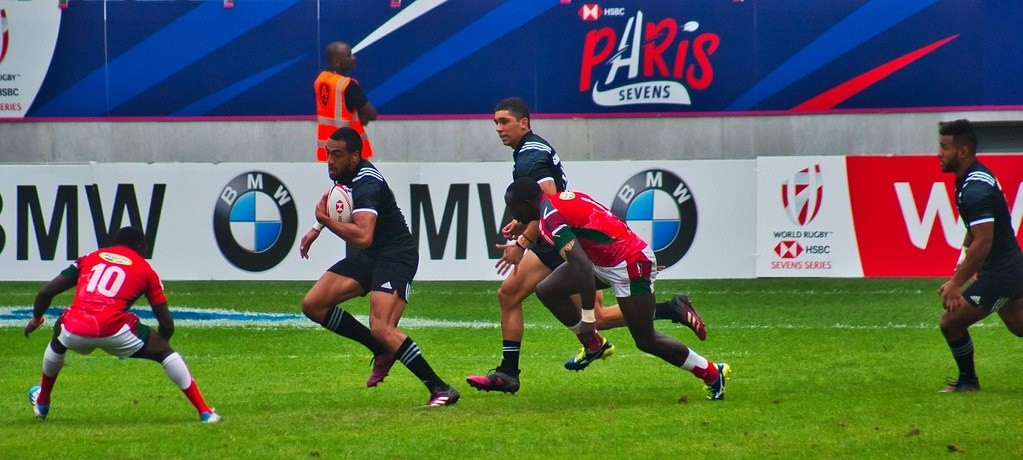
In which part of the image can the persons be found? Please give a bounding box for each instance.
[462,96,707,397]
[294,131,459,412]
[930,119,1023,395]
[21,228,223,425]
[312,41,378,165]
[490,179,731,400]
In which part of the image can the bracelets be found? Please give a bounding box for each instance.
[580,307,597,324]
[312,222,325,232]
[515,232,534,251]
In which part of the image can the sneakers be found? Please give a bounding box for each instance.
[29,385,51,420]
[422,383,460,407]
[200,412,223,423]
[669,293,707,341]
[938,377,980,393]
[564,337,615,372]
[465,365,521,395]
[702,363,733,401]
[367,352,397,387]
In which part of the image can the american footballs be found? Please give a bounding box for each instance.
[325,184,354,223]
